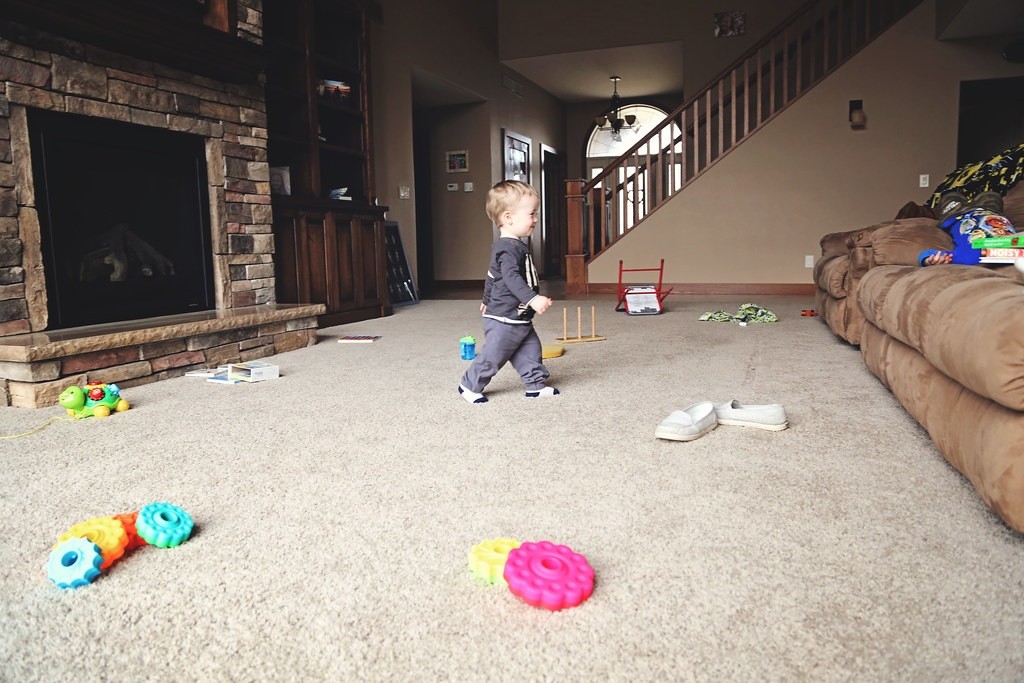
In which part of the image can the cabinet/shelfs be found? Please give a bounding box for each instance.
[262,0,395,329]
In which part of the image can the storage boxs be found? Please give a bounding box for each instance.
[227,361,279,382]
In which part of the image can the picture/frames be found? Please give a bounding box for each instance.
[445,150,469,174]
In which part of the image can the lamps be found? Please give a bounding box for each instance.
[849,100,864,126]
[592,76,635,133]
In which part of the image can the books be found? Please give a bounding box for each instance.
[338,336,381,343]
[972,230,1024,264]
[185,363,252,385]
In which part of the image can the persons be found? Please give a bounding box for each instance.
[271,174,287,195]
[717,15,736,36]
[918,192,1017,268]
[459,179,560,403]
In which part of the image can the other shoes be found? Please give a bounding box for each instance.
[654,401,718,441]
[715,398,789,431]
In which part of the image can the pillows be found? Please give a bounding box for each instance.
[895,201,934,219]
[868,224,955,265]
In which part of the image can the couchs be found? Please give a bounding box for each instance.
[813,175,1024,538]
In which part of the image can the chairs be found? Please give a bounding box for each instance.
[615,258,674,316]
